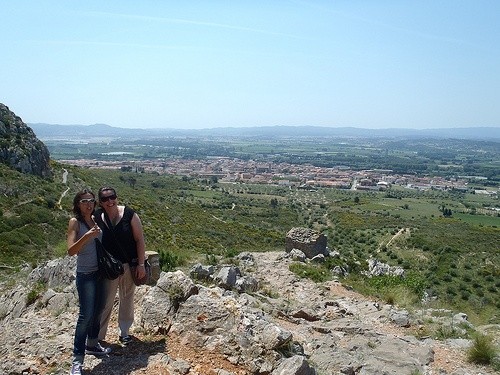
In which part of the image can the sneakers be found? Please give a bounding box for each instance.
[70,361,84,375]
[85,342,111,354]
[119,336,132,344]
[98,341,110,348]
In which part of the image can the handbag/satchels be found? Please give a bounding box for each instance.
[94,238,125,280]
[129,258,152,286]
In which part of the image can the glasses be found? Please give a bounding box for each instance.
[79,198,95,204]
[99,195,118,202]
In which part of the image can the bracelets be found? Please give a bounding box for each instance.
[138,263,145,267]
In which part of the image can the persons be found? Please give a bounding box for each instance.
[93,186,146,343]
[67,190,112,375]
